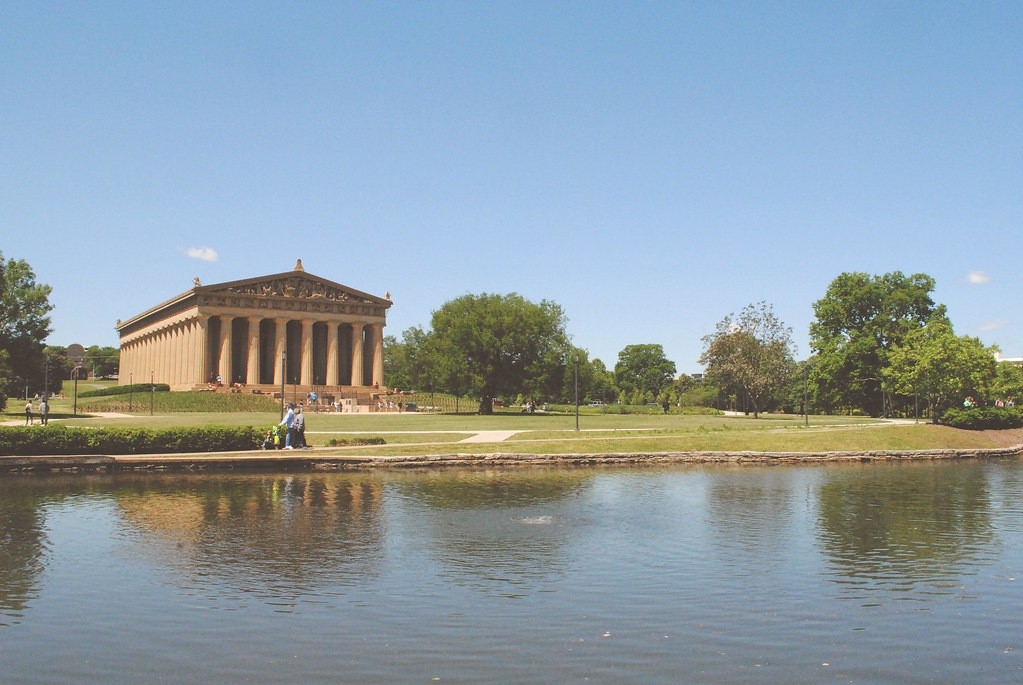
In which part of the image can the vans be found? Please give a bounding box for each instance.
[588,401,606,407]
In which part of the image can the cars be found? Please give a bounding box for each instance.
[495,401,509,407]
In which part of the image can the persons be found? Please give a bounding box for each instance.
[216,375,222,388]
[331,401,342,412]
[378,399,402,413]
[24,400,34,425]
[526,400,535,414]
[39,399,50,425]
[312,392,318,404]
[299,399,303,405]
[209,372,215,384]
[261,403,308,450]
[306,392,311,405]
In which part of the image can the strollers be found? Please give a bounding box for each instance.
[262,425,278,450]
[522,408,528,413]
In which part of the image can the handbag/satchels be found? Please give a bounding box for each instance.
[273,432,281,445]
